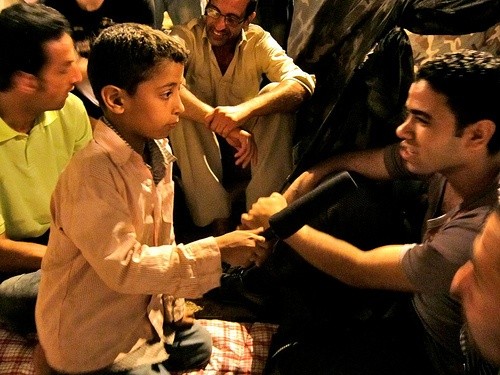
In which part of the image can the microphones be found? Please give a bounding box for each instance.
[221,171,359,272]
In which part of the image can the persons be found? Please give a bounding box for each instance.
[0,4,94,313]
[41,0,499,251]
[34,23,269,375]
[233,49,500,375]
[450,210,500,375]
